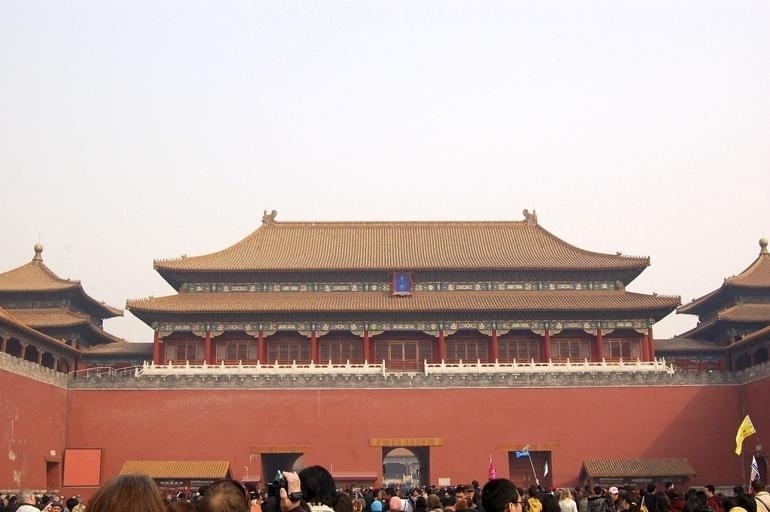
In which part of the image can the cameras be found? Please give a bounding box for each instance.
[267,476,288,500]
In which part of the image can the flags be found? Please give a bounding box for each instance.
[515,447,529,458]
[749,455,761,481]
[542,461,550,478]
[734,415,756,456]
[488,456,497,481]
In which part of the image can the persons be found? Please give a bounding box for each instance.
[1,465,770,512]
[397,274,408,291]
